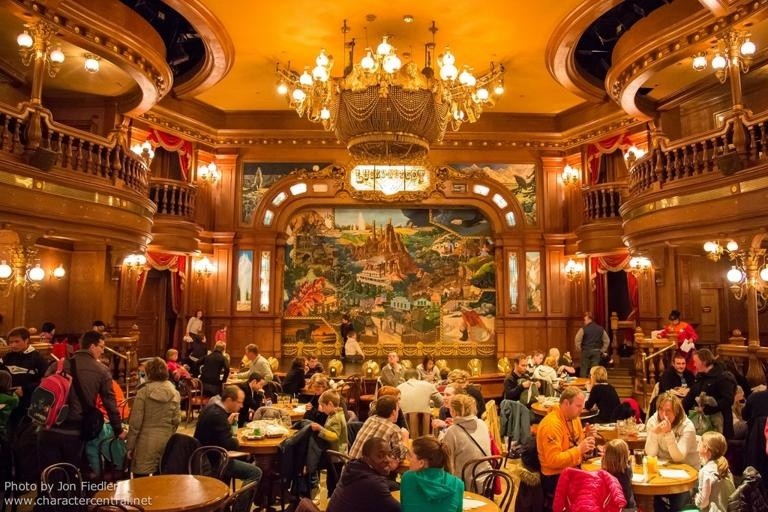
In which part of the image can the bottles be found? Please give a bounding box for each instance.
[231,420,238,439]
[584,426,594,458]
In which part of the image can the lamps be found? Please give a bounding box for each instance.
[275,19,505,158]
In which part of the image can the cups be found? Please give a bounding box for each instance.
[616,420,625,434]
[647,456,657,474]
[292,399,298,408]
[283,396,290,407]
[632,448,644,468]
[277,396,282,408]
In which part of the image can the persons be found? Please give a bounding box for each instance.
[1,309,768,512]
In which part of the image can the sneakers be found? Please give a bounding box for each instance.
[311,484,321,505]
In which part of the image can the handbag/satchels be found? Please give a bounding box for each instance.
[687,410,711,436]
[28,372,73,427]
[81,406,104,441]
